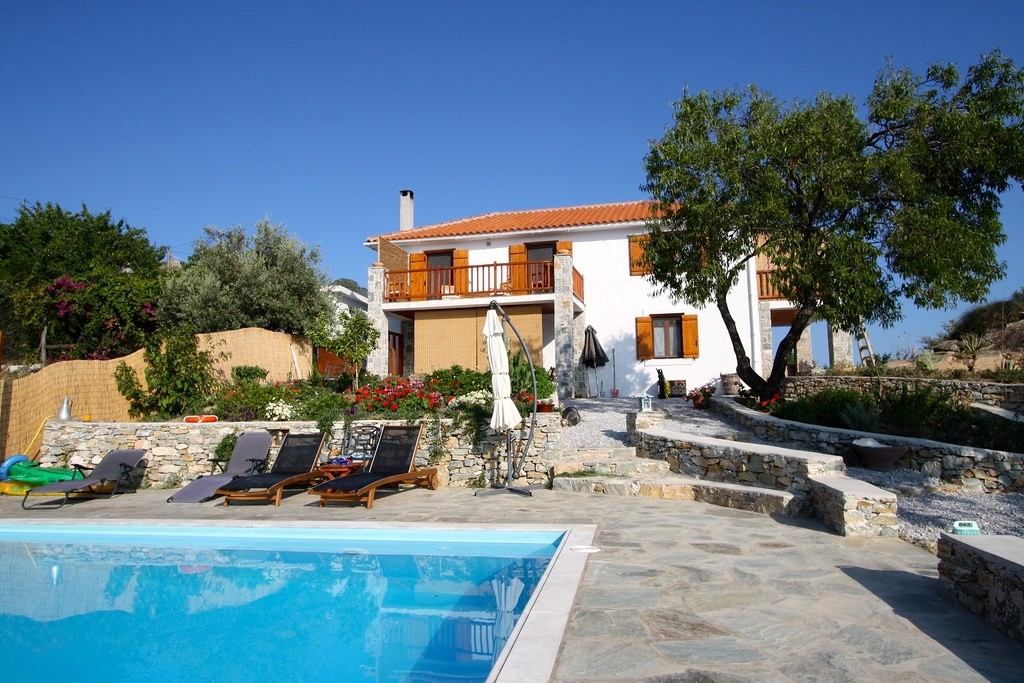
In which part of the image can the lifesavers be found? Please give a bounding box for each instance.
[185,414,217,423]
[0,455,30,482]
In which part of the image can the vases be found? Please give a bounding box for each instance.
[537,404,555,412]
[692,398,709,408]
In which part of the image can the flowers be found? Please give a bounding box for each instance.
[537,398,554,405]
[689,385,716,401]
[266,397,293,421]
[762,393,786,412]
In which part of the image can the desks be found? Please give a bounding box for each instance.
[316,462,362,481]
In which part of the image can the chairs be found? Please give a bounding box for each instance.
[305,424,439,509]
[21,448,148,511]
[215,429,328,507]
[166,429,272,504]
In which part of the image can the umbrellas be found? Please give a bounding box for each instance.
[583,325,609,399]
[475,299,538,498]
